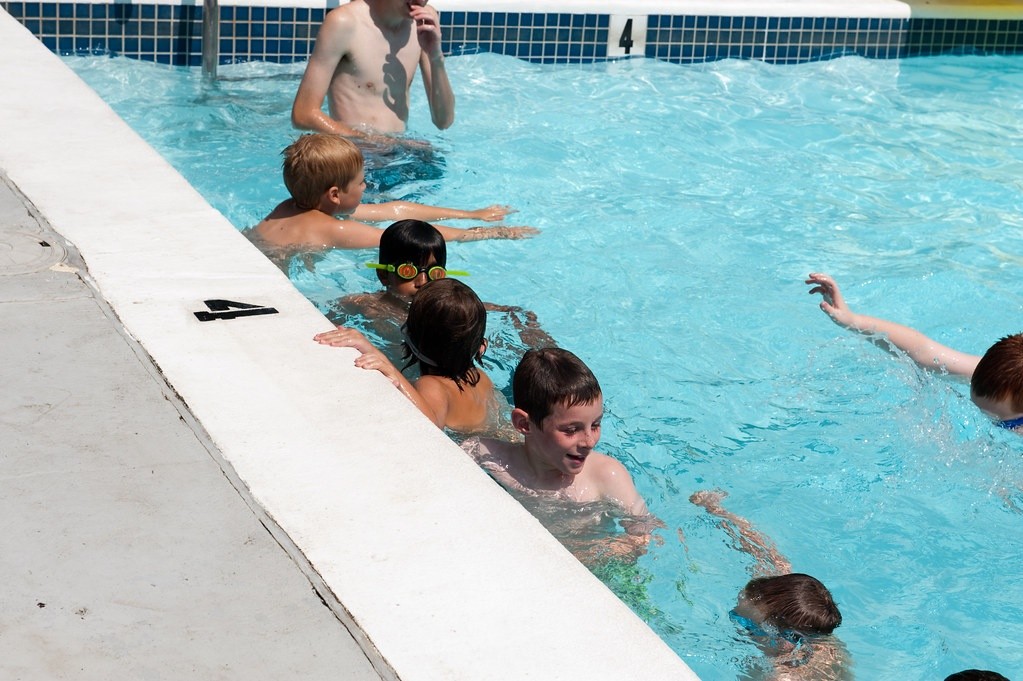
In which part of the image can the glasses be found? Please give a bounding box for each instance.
[389,260,447,282]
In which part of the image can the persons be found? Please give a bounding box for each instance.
[805,271,1023,443]
[689,487,858,680]
[942,669,1013,681]
[457,347,650,569]
[313,278,521,445]
[291,0,455,155]
[325,218,525,361]
[242,130,542,284]
